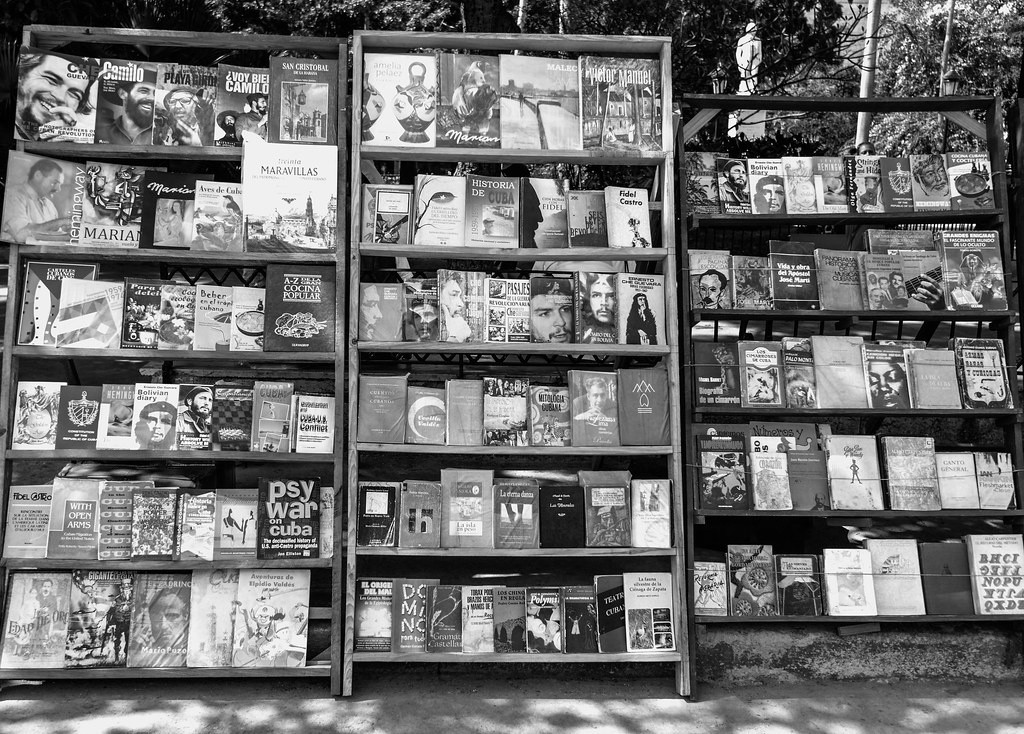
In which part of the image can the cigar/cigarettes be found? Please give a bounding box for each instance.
[58,111,76,127]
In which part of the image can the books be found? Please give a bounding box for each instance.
[685,152,1024,616]
[0,52,338,668]
[354,53,676,654]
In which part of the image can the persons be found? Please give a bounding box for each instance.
[132,586,187,664]
[857,142,875,156]
[720,162,748,202]
[179,387,213,446]
[12,582,39,656]
[440,274,471,343]
[754,174,785,211]
[870,363,907,408]
[135,401,177,450]
[412,297,438,341]
[736,23,762,94]
[581,278,615,331]
[361,286,382,341]
[3,48,268,248]
[573,372,617,420]
[24,579,57,658]
[533,281,575,344]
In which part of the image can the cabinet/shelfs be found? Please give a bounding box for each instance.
[674,93,1024,701]
[0,25,347,698]
[343,29,691,696]
